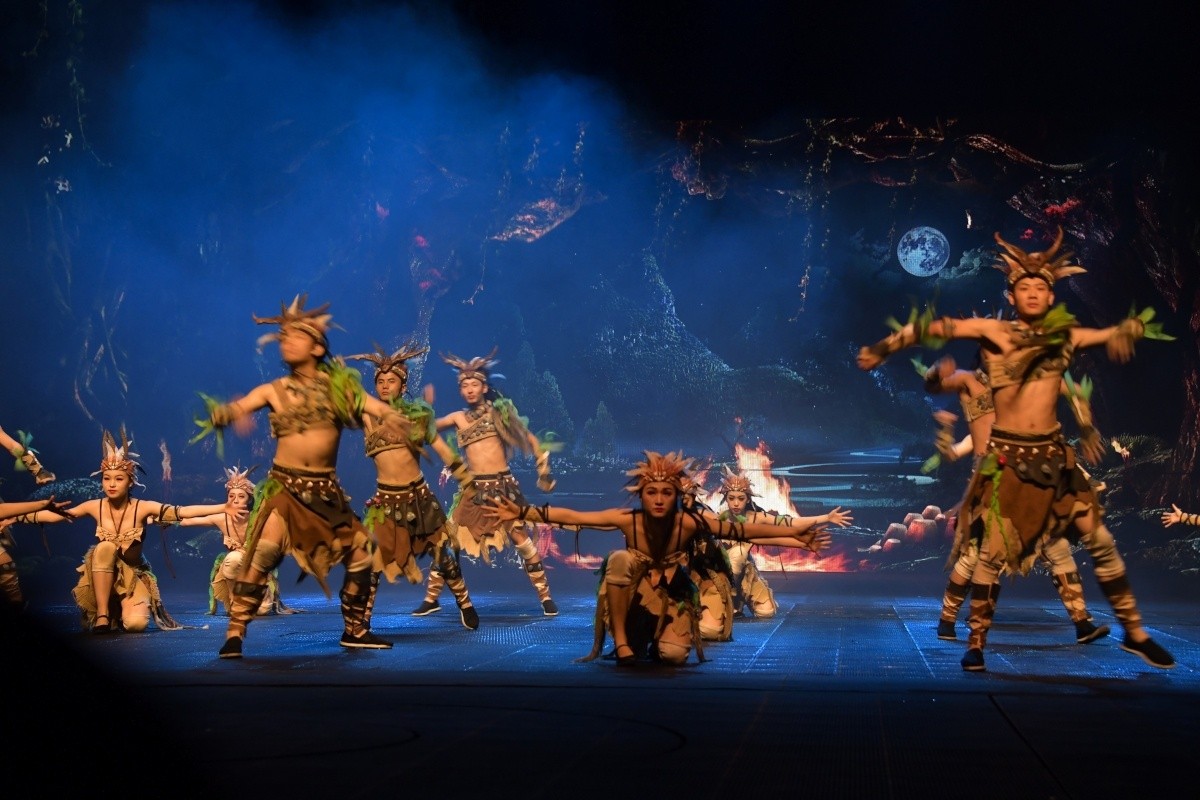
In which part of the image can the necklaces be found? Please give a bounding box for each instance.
[231,515,250,546]
[108,496,130,551]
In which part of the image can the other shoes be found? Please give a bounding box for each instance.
[542,599,559,616]
[615,643,638,666]
[410,599,443,616]
[338,631,393,650]
[218,636,243,658]
[1075,619,1108,642]
[90,615,112,634]
[937,619,957,640]
[460,607,480,629]
[961,647,985,670]
[1120,633,1175,669]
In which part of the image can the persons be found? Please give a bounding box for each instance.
[147,466,280,617]
[858,227,1175,670]
[212,295,417,658]
[480,449,855,670]
[0,425,250,637]
[1161,503,1200,529]
[331,345,482,632]
[410,345,562,616]
